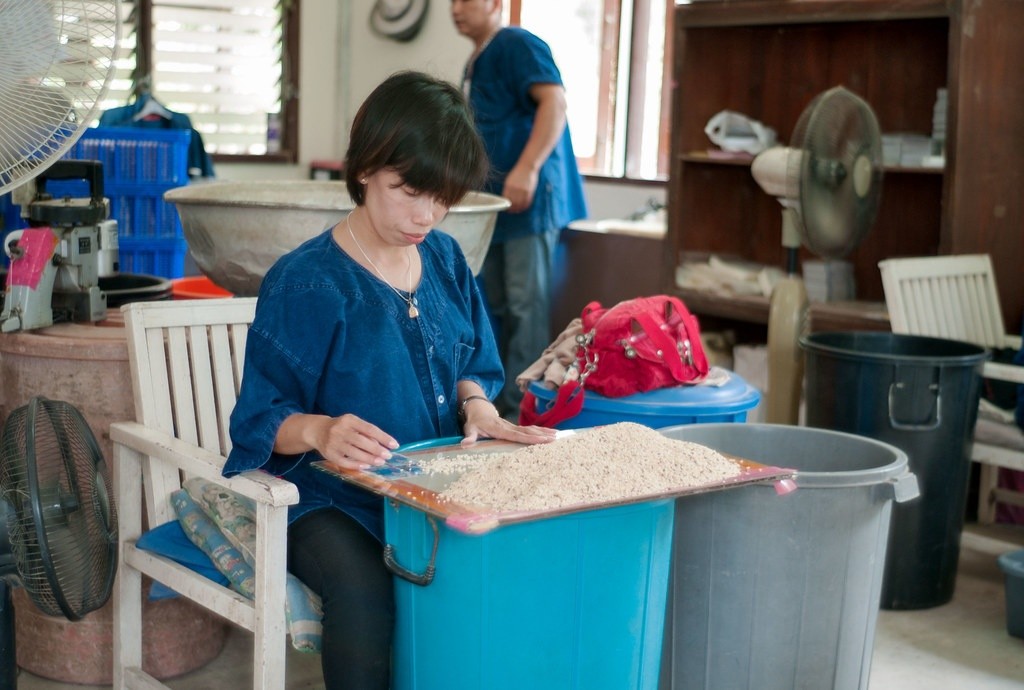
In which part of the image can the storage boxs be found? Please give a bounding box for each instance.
[733,343,811,427]
[36,125,194,279]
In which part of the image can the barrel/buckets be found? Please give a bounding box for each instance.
[383,436,675,690]
[98,274,172,308]
[527,366,760,432]
[172,277,234,300]
[797,331,993,610]
[659,423,918,690]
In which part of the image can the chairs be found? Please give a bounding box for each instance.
[111,296,323,690]
[879,254,1024,555]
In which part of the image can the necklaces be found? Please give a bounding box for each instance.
[346,213,421,320]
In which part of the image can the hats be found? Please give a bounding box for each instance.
[368,0,431,44]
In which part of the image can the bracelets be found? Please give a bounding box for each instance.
[457,394,496,408]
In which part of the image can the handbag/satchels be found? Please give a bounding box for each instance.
[575,292,710,399]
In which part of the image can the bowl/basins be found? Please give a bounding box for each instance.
[163,179,512,297]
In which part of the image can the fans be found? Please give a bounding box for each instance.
[0,393,121,690]
[751,85,884,426]
[0,0,124,197]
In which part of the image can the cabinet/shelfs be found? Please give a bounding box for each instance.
[664,0,1024,430]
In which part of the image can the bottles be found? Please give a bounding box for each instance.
[930,90,948,155]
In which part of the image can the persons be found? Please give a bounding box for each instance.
[221,69,558,689]
[448,0,589,416]
